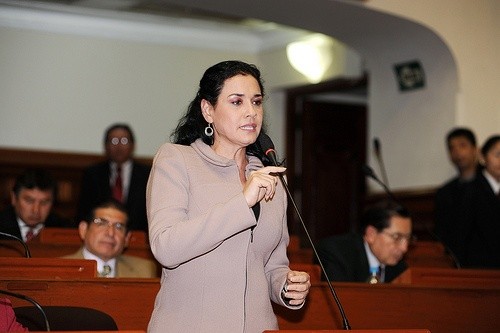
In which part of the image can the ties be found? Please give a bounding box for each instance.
[112,166,123,202]
[375,266,383,280]
[103,265,111,277]
[26,225,36,242]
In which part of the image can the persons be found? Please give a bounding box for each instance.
[77,125,151,230]
[141,61,310,333]
[58,199,157,280]
[440,135,500,269]
[320,206,413,283]
[0,176,57,241]
[433,128,487,234]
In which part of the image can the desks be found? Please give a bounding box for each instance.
[0,228,500,333]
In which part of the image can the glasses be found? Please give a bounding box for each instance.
[108,137,130,145]
[92,217,127,233]
[383,230,412,243]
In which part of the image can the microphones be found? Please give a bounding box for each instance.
[260,133,352,330]
[361,138,393,196]
